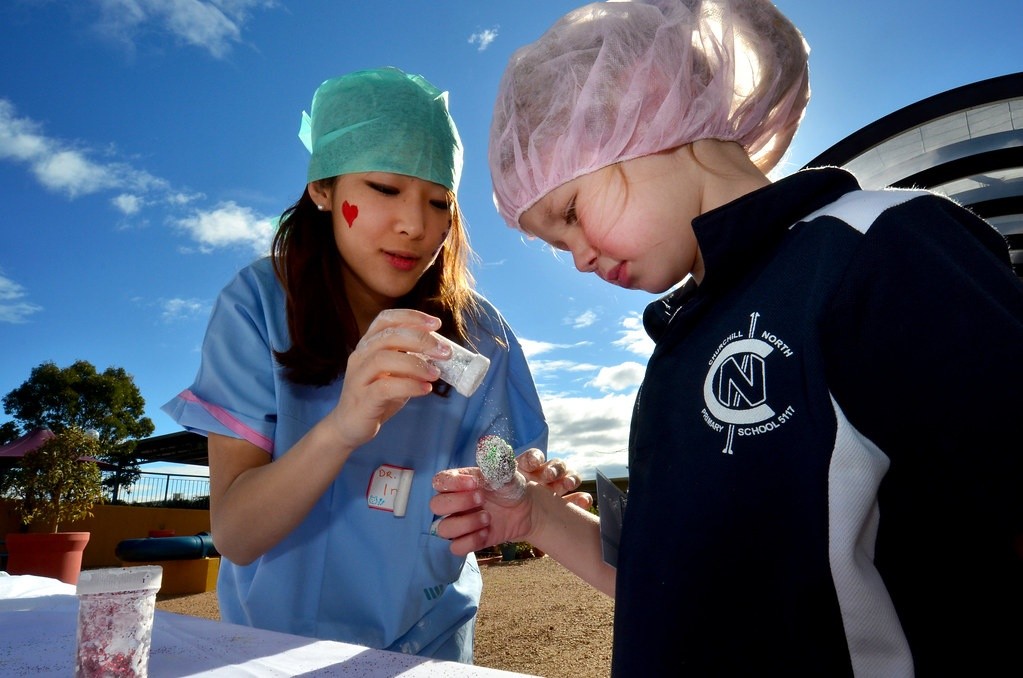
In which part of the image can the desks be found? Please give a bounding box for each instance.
[1,571,540,678]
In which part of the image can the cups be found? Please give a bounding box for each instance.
[73,564,163,678]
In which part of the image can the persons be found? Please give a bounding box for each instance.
[429,0,1023,678]
[160,66,593,666]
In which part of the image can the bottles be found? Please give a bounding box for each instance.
[407,332,491,397]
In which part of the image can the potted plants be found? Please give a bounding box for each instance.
[4,424,111,588]
